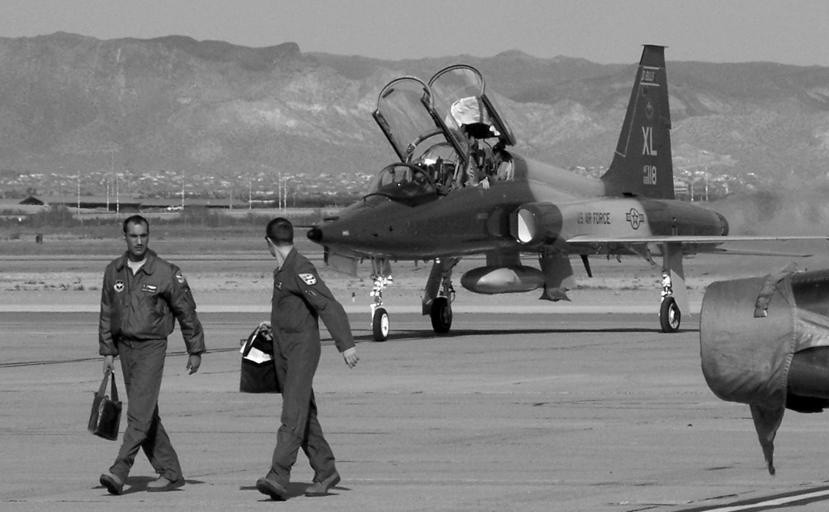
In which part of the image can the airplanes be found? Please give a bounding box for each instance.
[308,43,828,341]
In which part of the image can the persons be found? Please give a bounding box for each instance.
[99,215,206,495]
[256,217,360,501]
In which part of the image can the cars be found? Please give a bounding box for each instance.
[166,204,185,213]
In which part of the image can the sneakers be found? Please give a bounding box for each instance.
[258,476,287,500]
[147,472,185,491]
[305,471,340,496]
[100,472,124,494]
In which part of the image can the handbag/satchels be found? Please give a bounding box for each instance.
[239,324,282,393]
[87,391,123,441]
